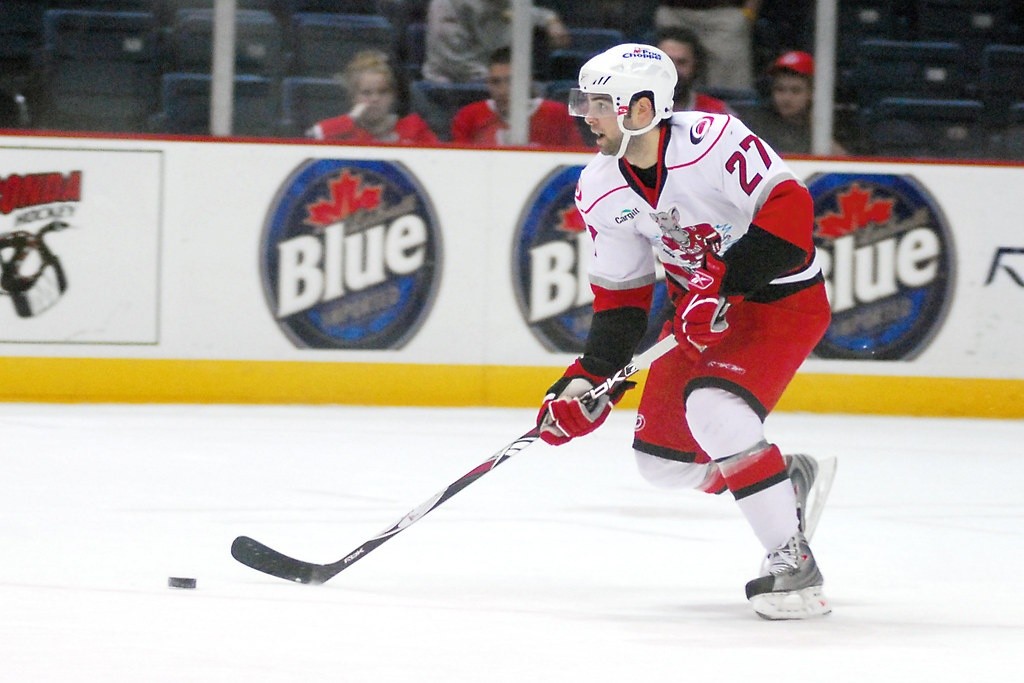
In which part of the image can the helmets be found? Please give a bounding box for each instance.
[567,43,678,136]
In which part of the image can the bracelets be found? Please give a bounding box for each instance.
[742,7,757,22]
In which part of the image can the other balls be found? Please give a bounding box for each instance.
[168,577,196,588]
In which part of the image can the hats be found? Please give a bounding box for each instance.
[776,50,813,77]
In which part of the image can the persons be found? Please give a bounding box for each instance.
[306,0,847,158]
[537,43,833,599]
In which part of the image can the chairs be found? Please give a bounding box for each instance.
[35,1,1024,162]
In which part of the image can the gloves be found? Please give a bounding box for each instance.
[672,250,730,360]
[536,357,637,446]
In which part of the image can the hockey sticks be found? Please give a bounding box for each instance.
[230,301,731,584]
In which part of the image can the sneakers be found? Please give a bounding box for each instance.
[745,507,832,620]
[785,452,836,546]
